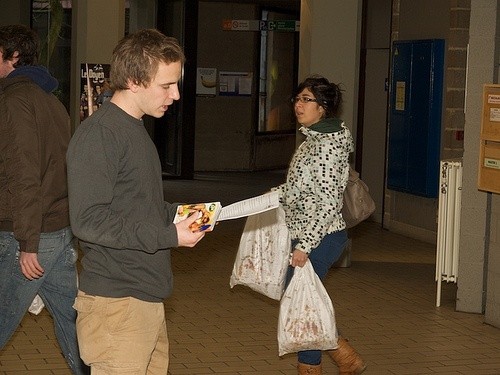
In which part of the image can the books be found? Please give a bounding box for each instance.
[173,202,221,232]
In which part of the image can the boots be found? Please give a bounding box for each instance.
[296,332,372,374]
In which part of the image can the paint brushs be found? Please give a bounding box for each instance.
[191,224,212,233]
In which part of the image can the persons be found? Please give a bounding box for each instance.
[66,27,205,375]
[0,24,91,375]
[264,75,368,375]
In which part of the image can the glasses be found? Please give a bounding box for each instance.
[291,95,320,104]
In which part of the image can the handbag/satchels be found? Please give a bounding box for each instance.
[229,183,290,302]
[339,164,377,226]
[276,250,340,359]
